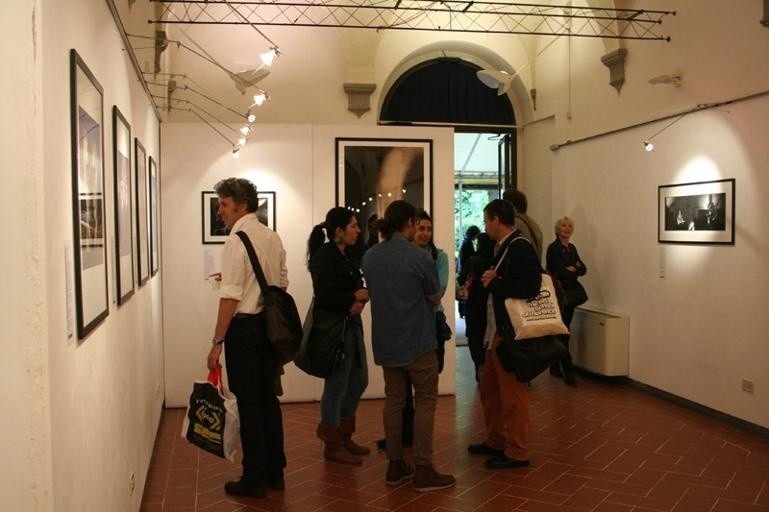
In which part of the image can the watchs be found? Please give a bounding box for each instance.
[212,336,223,344]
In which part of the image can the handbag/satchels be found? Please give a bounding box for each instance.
[553,275,589,311]
[178,363,242,465]
[502,271,573,341]
[290,293,345,383]
[456,274,473,303]
[251,285,305,370]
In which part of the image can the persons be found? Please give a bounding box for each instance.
[667,193,725,231]
[547,216,587,387]
[462,188,543,469]
[206,177,289,498]
[306,206,371,466]
[376,211,450,448]
[360,200,456,492]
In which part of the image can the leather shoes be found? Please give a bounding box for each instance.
[468,440,504,456]
[485,454,531,470]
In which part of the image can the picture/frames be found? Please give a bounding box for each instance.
[146,153,160,279]
[67,45,110,343]
[108,104,136,311]
[199,189,277,245]
[655,177,737,247]
[332,135,435,278]
[133,137,151,291]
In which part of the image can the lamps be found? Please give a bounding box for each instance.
[108,1,281,160]
[472,0,575,97]
[640,98,730,152]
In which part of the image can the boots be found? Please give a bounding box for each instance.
[563,358,578,387]
[549,361,562,380]
[340,414,371,455]
[385,455,415,487]
[412,463,458,494]
[316,423,362,465]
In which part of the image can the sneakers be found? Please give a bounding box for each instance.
[375,432,414,450]
[223,474,267,498]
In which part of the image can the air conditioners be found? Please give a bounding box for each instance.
[568,306,632,380]
